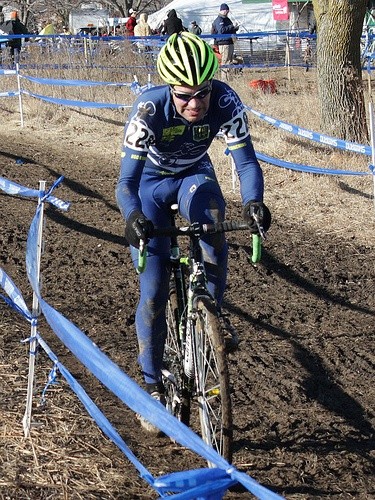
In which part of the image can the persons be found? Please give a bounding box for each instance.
[115,30,272,435]
[212,3,240,83]
[126,12,138,47]
[162,9,183,35]
[1,12,33,70]
[189,21,202,35]
[133,13,152,52]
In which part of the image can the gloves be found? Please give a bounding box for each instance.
[243,199,271,237]
[125,210,146,249]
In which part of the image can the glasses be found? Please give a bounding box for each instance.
[168,79,213,101]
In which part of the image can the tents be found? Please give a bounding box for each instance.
[147,0,375,54]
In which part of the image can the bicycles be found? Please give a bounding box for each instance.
[133,202,266,475]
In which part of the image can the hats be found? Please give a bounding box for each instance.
[220,3,229,10]
[191,20,196,24]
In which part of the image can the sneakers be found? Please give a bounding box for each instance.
[218,313,239,353]
[136,390,165,436]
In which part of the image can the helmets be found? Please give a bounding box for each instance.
[156,31,219,87]
